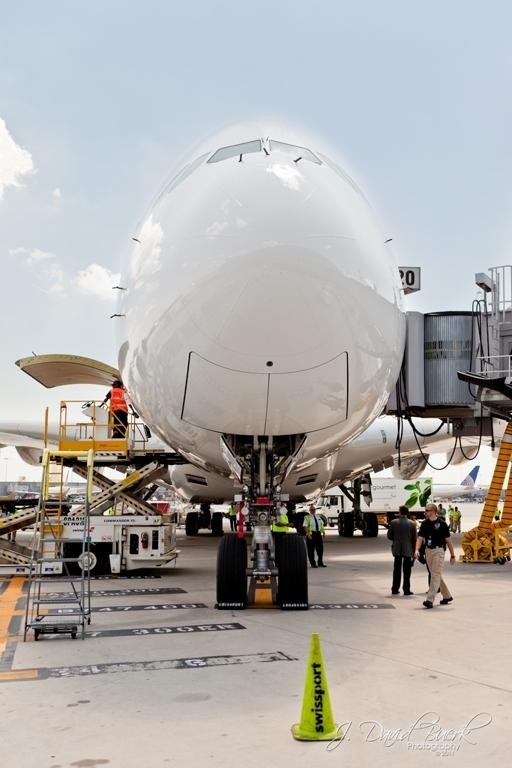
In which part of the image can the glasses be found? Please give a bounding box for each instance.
[426,509,434,512]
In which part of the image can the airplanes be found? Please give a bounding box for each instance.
[432,466,490,502]
[16,469,103,498]
[0,129,511,610]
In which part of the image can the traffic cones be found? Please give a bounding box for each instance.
[292,634,343,742]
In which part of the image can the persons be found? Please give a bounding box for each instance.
[303,505,327,568]
[386,504,461,609]
[228,503,238,532]
[99,380,129,438]
[273,514,298,533]
[494,506,501,521]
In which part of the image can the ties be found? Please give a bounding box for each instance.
[314,516,318,532]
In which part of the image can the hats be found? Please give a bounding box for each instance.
[111,381,123,385]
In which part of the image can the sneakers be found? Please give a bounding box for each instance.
[311,564,327,568]
[440,597,453,604]
[393,592,414,595]
[423,600,433,608]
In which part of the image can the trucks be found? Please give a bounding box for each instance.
[316,477,433,528]
